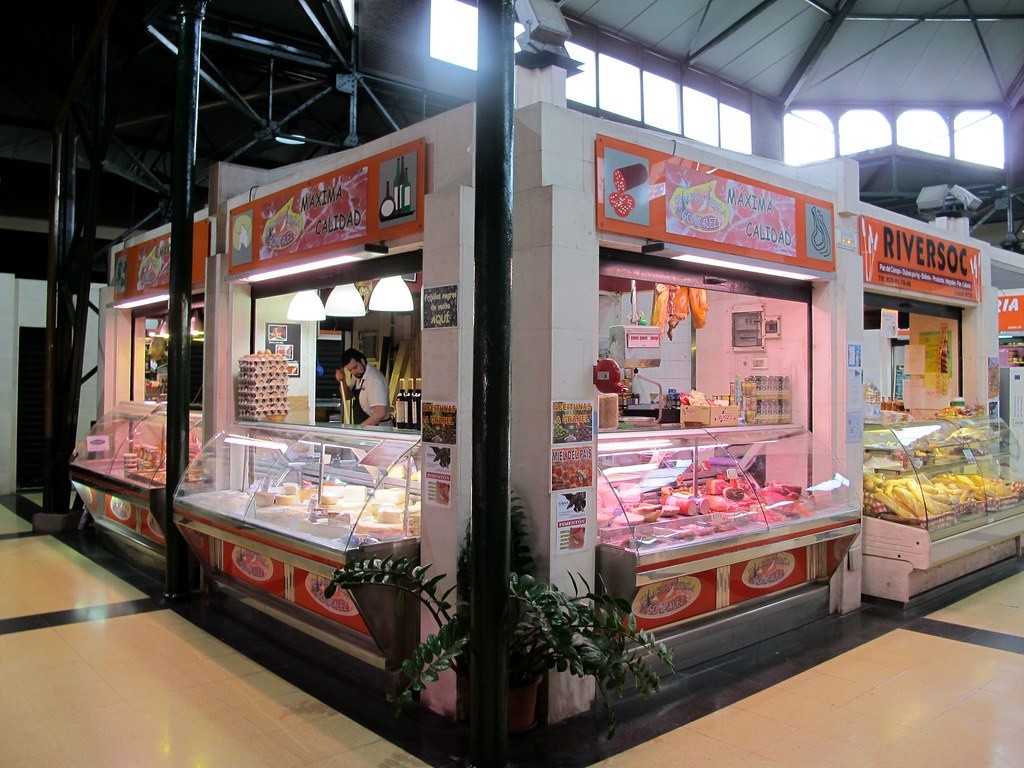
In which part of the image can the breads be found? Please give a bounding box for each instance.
[863,473,1010,519]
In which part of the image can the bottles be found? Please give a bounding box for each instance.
[395,378,407,429]
[756,398,791,416]
[404,377,415,429]
[879,396,899,424]
[744,375,791,392]
[411,377,422,431]
[863,380,883,425]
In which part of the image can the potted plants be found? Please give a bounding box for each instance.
[323,488,678,743]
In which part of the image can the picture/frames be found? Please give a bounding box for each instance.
[265,322,300,377]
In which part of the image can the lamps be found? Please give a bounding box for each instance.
[369,276,414,312]
[160,316,170,338]
[287,290,326,321]
[155,319,164,336]
[191,309,204,335]
[325,283,365,316]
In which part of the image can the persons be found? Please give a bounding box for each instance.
[335,348,394,428]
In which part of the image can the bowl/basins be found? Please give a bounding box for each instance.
[265,414,287,422]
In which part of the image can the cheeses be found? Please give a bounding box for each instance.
[253,482,421,541]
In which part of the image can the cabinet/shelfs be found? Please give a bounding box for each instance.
[862,417,1023,607]
